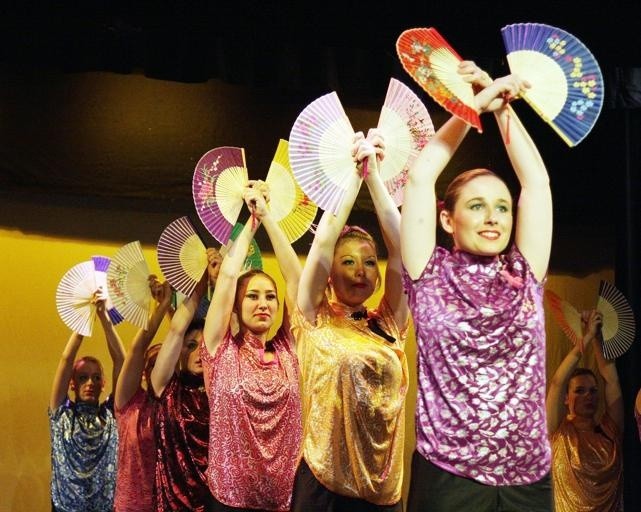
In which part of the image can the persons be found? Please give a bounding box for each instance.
[145,246,225,512]
[394,57,555,511]
[46,286,125,511]
[291,127,411,512]
[112,272,176,511]
[544,307,626,510]
[197,178,305,511]
